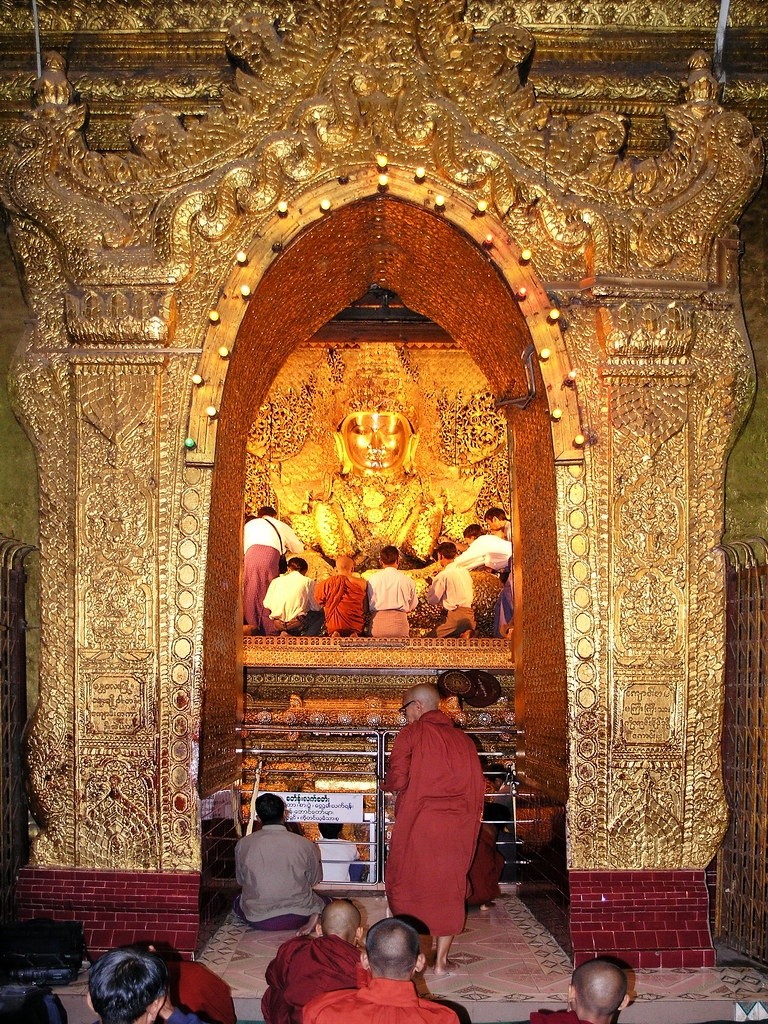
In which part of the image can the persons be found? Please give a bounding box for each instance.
[166,958,237,1023]
[453,525,514,637]
[528,960,629,1024]
[302,918,460,1024]
[430,543,476,638]
[465,822,503,911]
[243,506,305,636]
[262,558,321,637]
[87,947,204,1024]
[233,793,333,936]
[260,900,366,1024]
[313,823,364,882]
[365,546,418,638]
[485,804,518,880]
[484,507,512,541]
[313,558,368,638]
[300,345,447,568]
[488,766,513,806]
[388,683,482,976]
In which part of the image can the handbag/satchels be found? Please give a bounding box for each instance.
[491,569,509,583]
[0,918,84,969]
[278,554,287,574]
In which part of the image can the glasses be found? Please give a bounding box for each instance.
[398,700,416,715]
